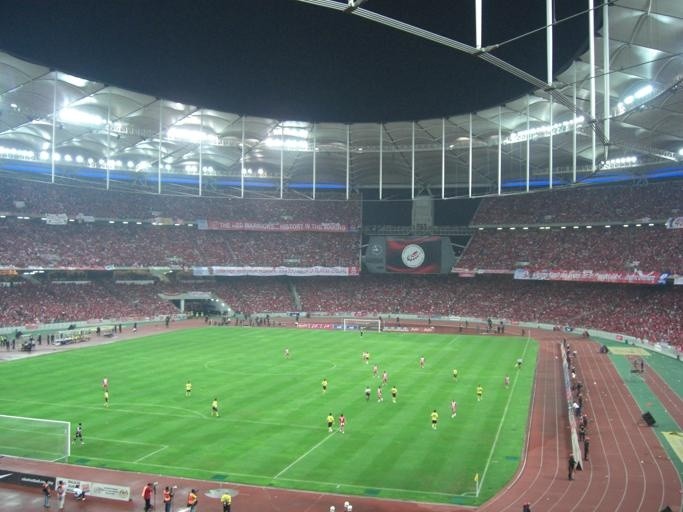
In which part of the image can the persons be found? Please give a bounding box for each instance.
[320,352,399,433]
[562,336,590,481]
[142,482,232,512]
[42,477,87,509]
[2,177,681,357]
[186,380,192,397]
[102,377,110,407]
[73,423,86,444]
[212,398,223,416]
[419,356,523,431]
